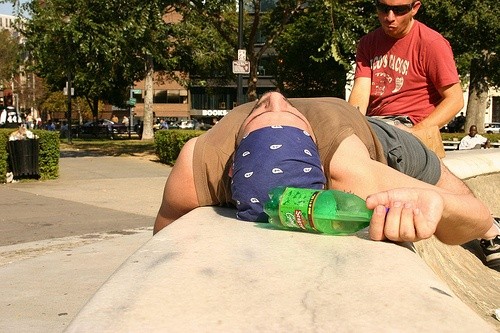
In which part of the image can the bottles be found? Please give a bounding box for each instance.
[263,186,391,236]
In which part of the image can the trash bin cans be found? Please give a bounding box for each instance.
[7,127,41,180]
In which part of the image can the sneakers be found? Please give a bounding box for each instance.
[480,218,500,268]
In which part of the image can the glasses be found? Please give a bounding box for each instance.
[376,1,417,15]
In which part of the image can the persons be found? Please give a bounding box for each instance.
[20,107,219,139]
[458,125,492,150]
[348,0,464,162]
[153,91,500,269]
[0,100,23,128]
[455,111,465,132]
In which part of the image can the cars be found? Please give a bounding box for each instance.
[84,119,114,126]
[485,123,500,132]
[172,120,195,129]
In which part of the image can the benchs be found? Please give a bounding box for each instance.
[110,126,137,140]
[79,125,109,140]
[442,138,500,150]
